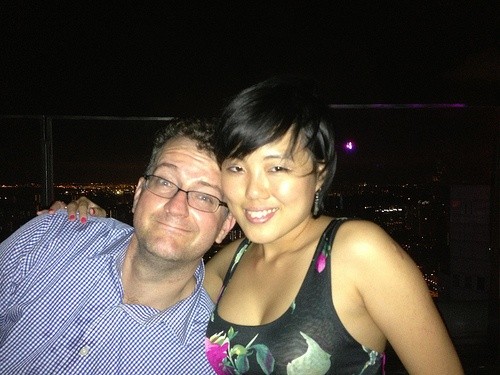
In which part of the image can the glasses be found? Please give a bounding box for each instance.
[144,174,228,214]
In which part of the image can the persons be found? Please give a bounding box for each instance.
[35,79,469,375]
[0,114,242,375]
